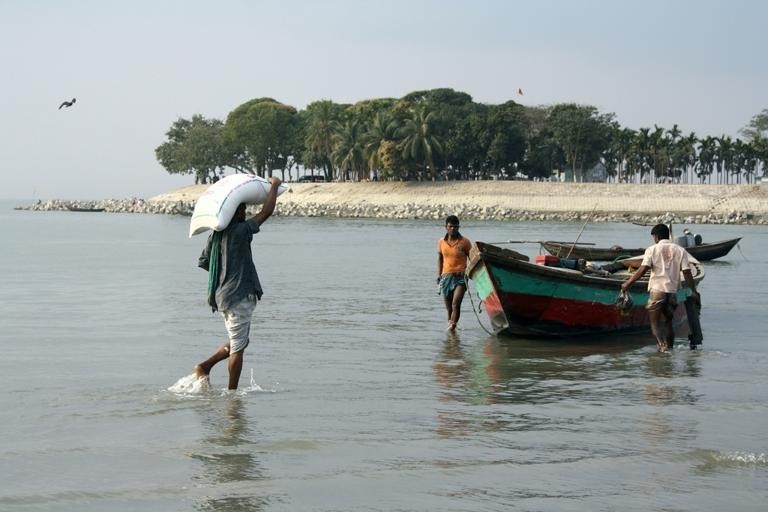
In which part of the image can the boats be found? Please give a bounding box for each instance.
[465,240,705,340]
[537,237,741,262]
[67,205,104,212]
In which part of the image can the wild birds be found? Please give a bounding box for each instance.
[519,88,524,96]
[58,98,76,110]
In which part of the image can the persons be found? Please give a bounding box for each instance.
[212,173,219,184]
[435,215,472,331]
[194,176,282,390]
[621,223,698,353]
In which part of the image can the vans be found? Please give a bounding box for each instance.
[299,175,324,182]
